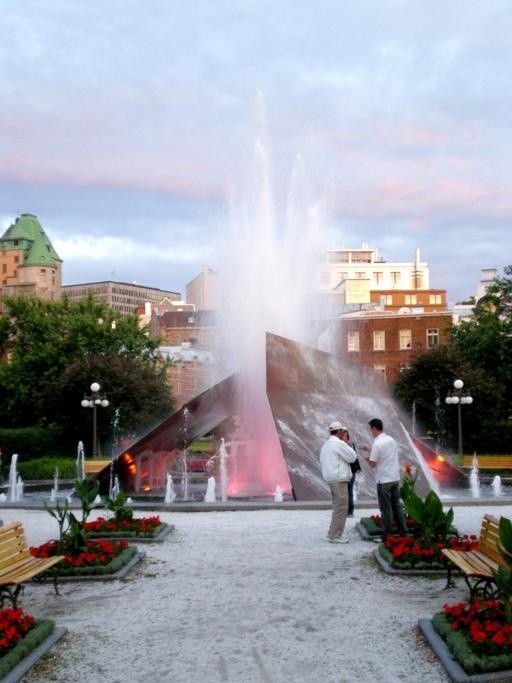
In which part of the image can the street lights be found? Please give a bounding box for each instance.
[445,379,474,469]
[81,381,109,464]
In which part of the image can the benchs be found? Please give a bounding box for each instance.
[458,455,512,476]
[84,461,111,474]
[0,521,65,610]
[441,515,510,602]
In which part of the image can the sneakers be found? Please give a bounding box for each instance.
[325,534,350,544]
[373,538,383,542]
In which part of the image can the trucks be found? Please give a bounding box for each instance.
[203,440,247,477]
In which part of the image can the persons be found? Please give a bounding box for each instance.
[320,421,361,544]
[357,418,407,544]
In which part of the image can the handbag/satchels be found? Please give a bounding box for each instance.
[350,459,361,474]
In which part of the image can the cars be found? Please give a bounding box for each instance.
[182,451,211,472]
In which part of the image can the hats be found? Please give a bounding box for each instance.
[329,421,347,432]
